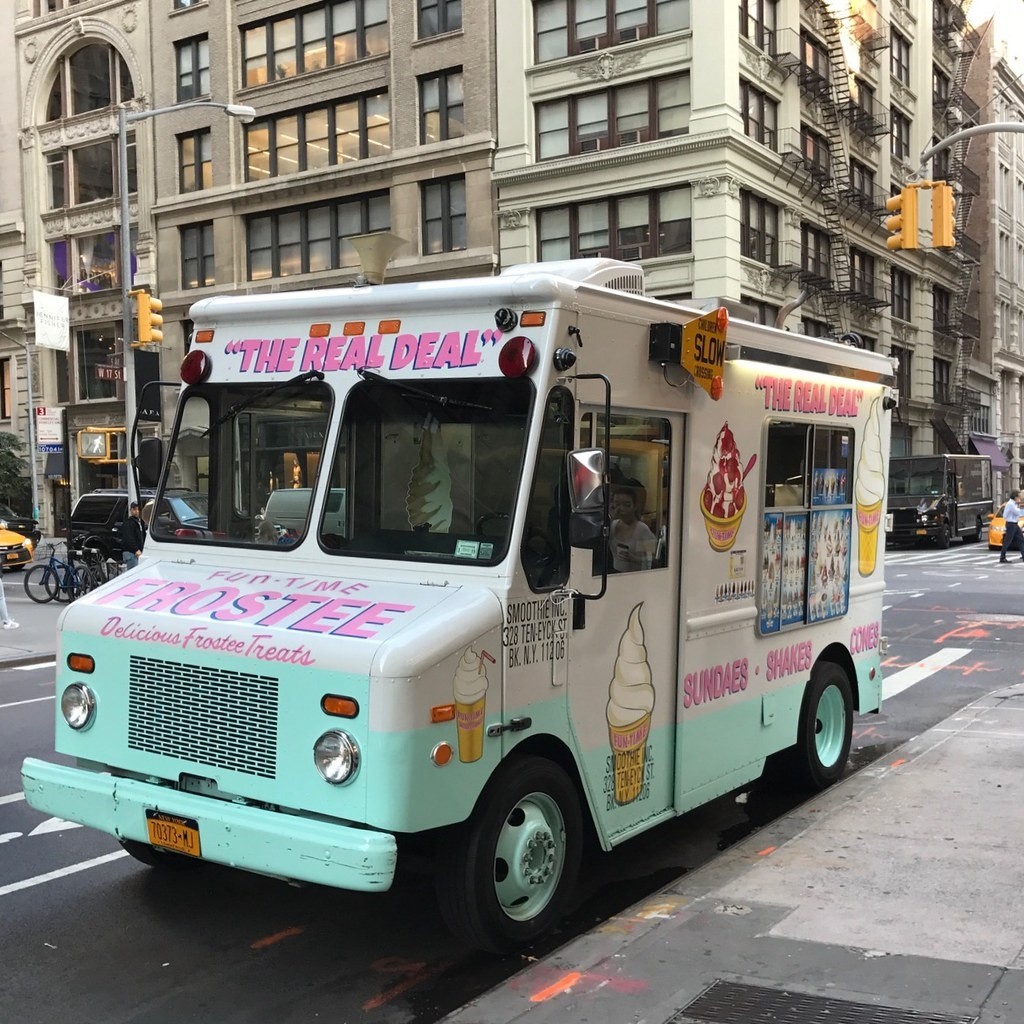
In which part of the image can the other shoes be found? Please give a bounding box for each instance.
[1000,559,1012,563]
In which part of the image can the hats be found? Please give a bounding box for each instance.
[130,501,138,508]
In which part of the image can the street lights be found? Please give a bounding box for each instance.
[116,103,256,518]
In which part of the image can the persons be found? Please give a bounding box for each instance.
[1000,490,1024,563]
[608,477,658,572]
[121,502,146,570]
[0,578,20,629]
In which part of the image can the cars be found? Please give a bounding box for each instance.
[0,522,35,573]
[0,500,42,550]
[987,503,1024,548]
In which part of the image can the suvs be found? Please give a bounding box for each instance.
[65,487,254,581]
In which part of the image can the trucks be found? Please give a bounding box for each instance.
[885,453,995,549]
[19,256,899,957]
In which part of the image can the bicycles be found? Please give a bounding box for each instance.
[22,531,129,606]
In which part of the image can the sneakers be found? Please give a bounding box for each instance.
[5,619,19,629]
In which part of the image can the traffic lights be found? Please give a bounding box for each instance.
[883,188,921,252]
[76,426,110,459]
[932,182,956,248]
[136,292,164,346]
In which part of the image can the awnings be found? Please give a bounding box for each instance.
[969,438,1010,471]
[45,453,126,480]
